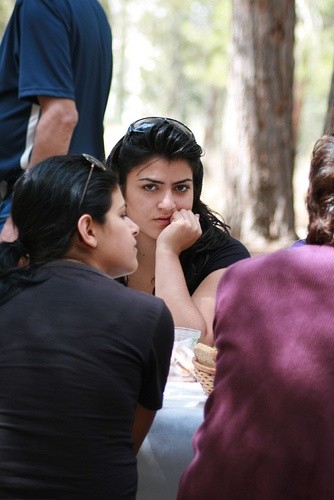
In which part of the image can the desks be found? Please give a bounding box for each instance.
[136,381,210,500]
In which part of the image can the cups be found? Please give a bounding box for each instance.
[170,327,202,377]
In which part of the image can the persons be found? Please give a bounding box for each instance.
[105,117,251,349]
[1,0,114,278]
[0,152,173,500]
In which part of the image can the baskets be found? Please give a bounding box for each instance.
[192,357,216,395]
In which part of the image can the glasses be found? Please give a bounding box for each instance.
[78,152,107,211]
[120,117,194,157]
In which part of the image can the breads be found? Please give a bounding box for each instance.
[192,343,217,369]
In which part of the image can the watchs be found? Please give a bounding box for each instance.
[175,133,334,500]
